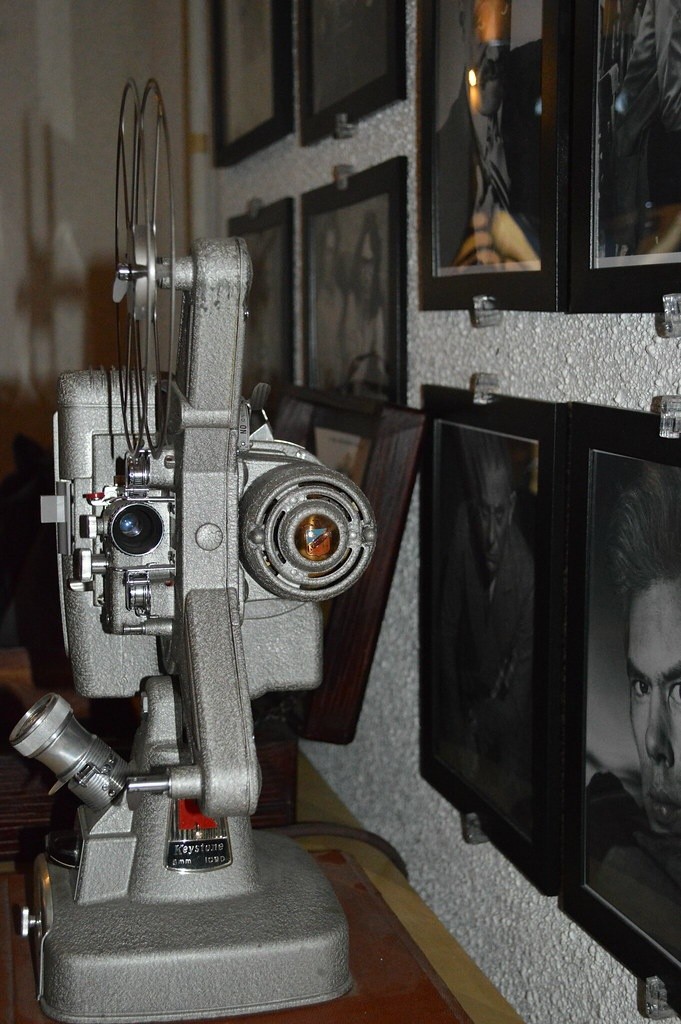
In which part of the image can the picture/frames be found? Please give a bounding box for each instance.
[298,0,407,146]
[563,0,678,313]
[282,405,423,745]
[415,387,552,891]
[550,400,681,1020]
[226,194,296,399]
[302,156,409,412]
[210,0,294,171]
[418,0,564,313]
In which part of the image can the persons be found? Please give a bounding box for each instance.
[314,218,348,394]
[430,0,551,267]
[586,461,680,963]
[335,211,387,393]
[436,448,538,830]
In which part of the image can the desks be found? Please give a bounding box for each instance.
[0,640,523,1024]
